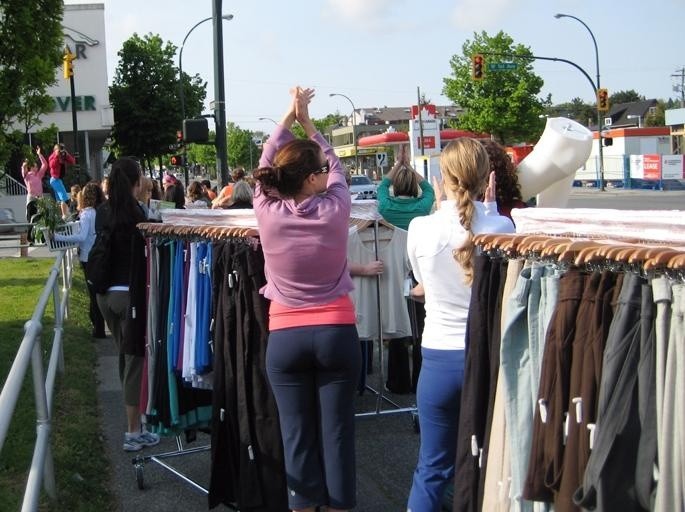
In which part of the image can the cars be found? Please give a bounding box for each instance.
[350,175,378,199]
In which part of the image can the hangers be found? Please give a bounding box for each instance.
[471,231,684,284]
[135,223,261,252]
[349,213,395,245]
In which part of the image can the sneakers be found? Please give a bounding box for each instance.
[123,432,160,450]
[92,327,105,338]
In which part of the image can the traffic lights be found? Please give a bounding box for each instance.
[473,54,483,82]
[63,55,75,79]
[172,156,181,166]
[176,131,183,148]
[597,90,608,111]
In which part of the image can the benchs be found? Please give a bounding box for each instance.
[1,223,37,256]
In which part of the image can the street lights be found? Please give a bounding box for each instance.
[555,13,605,190]
[259,118,277,126]
[179,15,233,187]
[330,93,360,174]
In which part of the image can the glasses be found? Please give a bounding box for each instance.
[315,159,330,176]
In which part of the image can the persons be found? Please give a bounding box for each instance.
[61,168,253,223]
[49,143,76,221]
[253,84,364,511]
[49,180,108,339]
[21,145,48,247]
[86,156,162,451]
[405,135,517,512]
[376,142,435,230]
[408,139,528,303]
[347,258,385,278]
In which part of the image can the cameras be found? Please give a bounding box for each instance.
[60,143,67,158]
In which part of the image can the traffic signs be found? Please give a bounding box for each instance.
[489,63,518,71]
